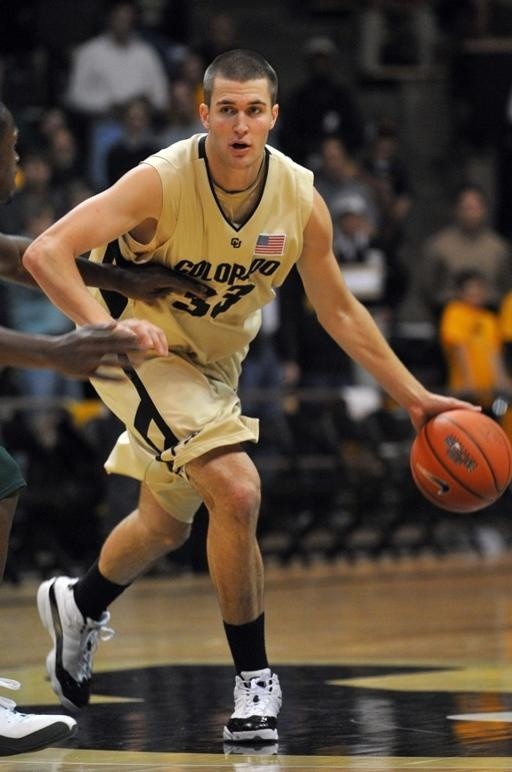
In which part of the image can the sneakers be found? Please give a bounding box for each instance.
[2,695,80,753]
[36,576,102,711]
[220,664,284,743]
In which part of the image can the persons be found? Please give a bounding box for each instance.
[1,103,210,765]
[17,46,483,744]
[1,0,510,595]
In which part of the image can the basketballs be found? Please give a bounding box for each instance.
[409,407,511,515]
